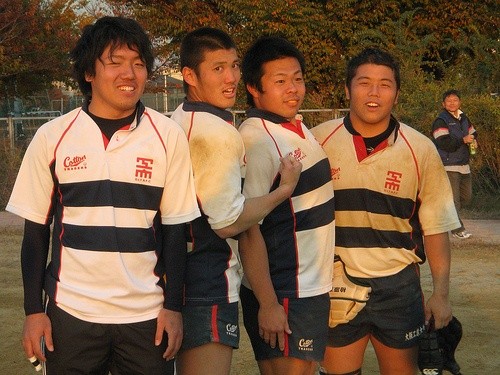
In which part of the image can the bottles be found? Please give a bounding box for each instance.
[469,135,477,154]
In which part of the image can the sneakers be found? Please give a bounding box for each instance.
[451,230,472,240]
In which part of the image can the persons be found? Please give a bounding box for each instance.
[169,26,302,375]
[431,91,476,240]
[308,48,452,375]
[238,34,334,375]
[5,15,202,375]
